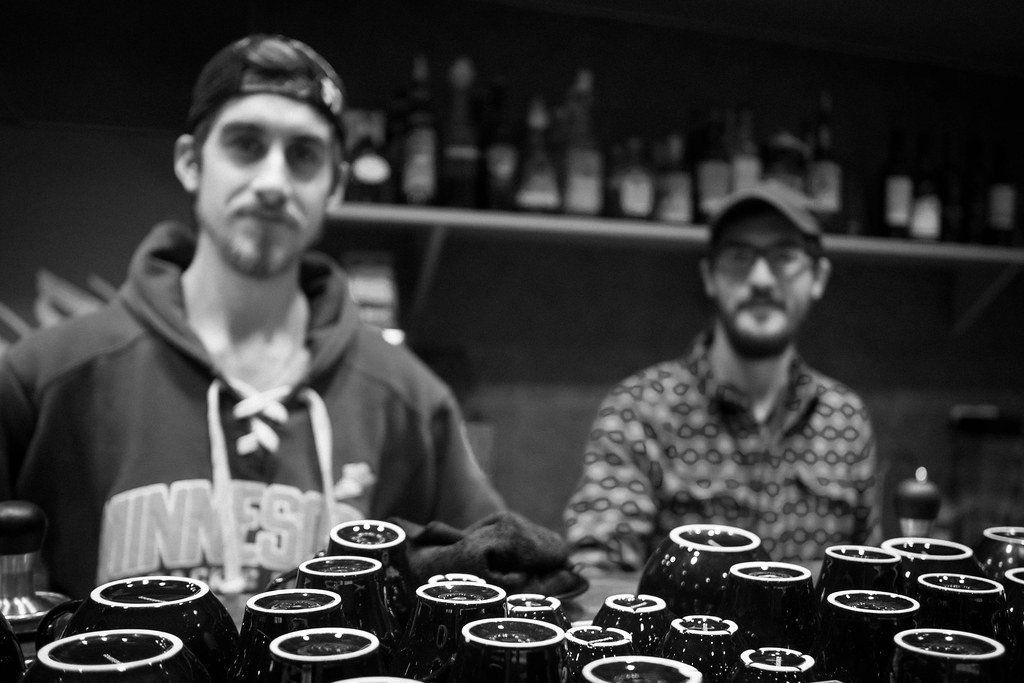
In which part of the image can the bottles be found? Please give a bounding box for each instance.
[348,50,1020,238]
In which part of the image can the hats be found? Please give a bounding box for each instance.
[710,178,824,249]
[185,34,347,146]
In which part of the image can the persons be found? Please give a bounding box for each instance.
[1,35,509,636]
[554,178,886,623]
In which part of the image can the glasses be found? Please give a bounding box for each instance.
[714,245,817,274]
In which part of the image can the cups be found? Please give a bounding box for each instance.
[566,524,819,683]
[814,526,1024,683]
[238,520,427,683]
[0,576,242,683]
[394,572,571,683]
